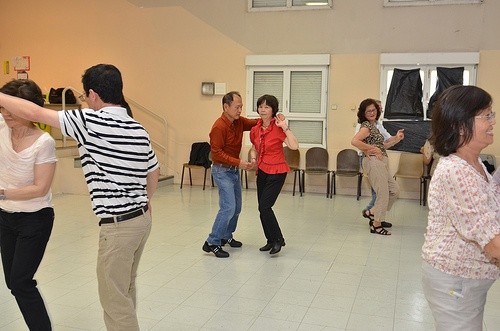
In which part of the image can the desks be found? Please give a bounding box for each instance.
[43,103,82,142]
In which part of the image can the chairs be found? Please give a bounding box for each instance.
[181,142,214,190]
[244,149,257,189]
[284,146,300,196]
[300,147,330,198]
[392,151,425,206]
[330,149,361,200]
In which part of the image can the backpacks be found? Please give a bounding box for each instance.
[187,142,212,169]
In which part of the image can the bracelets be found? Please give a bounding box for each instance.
[283,128,289,133]
[0,189,5,200]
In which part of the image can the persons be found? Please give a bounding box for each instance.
[351,98,405,235]
[248,94,299,254]
[202,92,285,258]
[423,139,441,176]
[0,63,160,331]
[422,85,500,331]
[0,79,59,331]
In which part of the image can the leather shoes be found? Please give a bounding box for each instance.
[270,238,285,254]
[260,241,273,251]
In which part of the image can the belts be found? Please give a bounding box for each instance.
[365,155,376,157]
[100,204,148,223]
[213,164,238,169]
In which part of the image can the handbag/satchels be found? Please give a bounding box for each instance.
[49,88,76,104]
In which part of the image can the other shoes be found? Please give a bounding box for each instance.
[362,209,366,213]
[369,219,392,227]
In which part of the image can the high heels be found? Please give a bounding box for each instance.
[362,208,374,226]
[370,225,391,235]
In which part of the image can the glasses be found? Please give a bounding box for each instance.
[78,88,98,102]
[366,108,376,114]
[475,111,496,120]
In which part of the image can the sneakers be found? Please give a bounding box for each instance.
[202,241,229,257]
[221,238,242,247]
